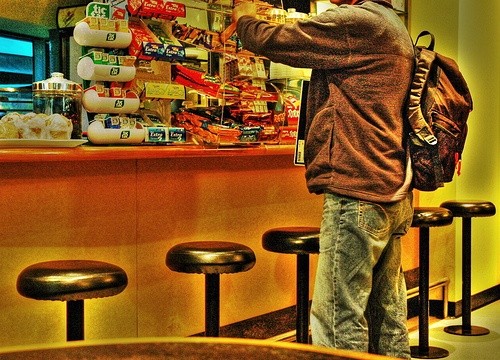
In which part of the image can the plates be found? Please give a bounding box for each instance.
[0,139,88,147]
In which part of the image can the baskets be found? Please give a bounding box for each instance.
[215,75,286,141]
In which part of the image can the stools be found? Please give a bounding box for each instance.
[15,202,496,359]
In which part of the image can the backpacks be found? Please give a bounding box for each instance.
[353,0,474,191]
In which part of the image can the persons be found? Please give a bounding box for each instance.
[233,0,414,360]
